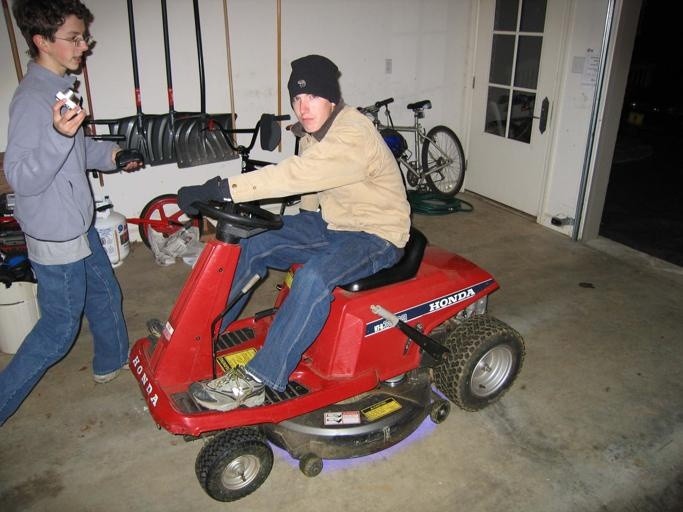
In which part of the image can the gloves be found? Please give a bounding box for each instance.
[177,176,223,216]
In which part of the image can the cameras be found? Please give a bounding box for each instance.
[55,88,81,114]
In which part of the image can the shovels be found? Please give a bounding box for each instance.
[119,0,239,168]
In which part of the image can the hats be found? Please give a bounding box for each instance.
[287,55,339,111]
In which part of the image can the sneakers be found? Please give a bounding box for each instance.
[190,363,266,412]
[146,318,165,339]
[92,348,131,384]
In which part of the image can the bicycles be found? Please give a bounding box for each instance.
[123,116,291,250]
[359,99,466,200]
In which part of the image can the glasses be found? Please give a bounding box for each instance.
[55,35,94,48]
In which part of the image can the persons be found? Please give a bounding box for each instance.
[0,0,146,429]
[146,53,414,414]
